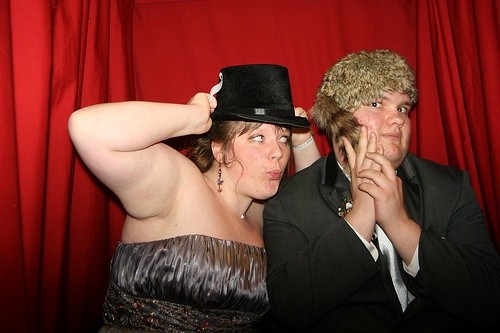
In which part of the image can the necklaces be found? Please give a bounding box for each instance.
[221,192,245,220]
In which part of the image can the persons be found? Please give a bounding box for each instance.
[263,50,500,333]
[68,64,321,333]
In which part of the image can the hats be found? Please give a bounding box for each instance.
[211,64,313,128]
[309,48,418,170]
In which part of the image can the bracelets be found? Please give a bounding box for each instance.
[291,134,314,152]
